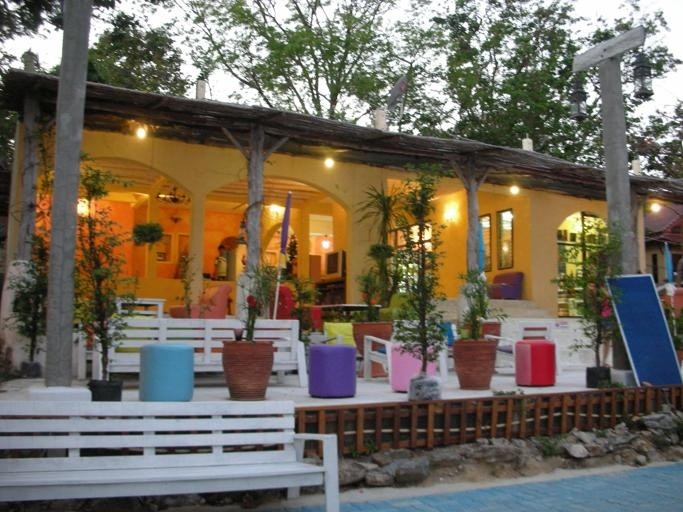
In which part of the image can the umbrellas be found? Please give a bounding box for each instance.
[664,242,676,340]
[477,223,486,320]
[273,190,293,318]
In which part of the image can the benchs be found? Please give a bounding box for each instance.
[0,400,340,512]
[91,318,308,388]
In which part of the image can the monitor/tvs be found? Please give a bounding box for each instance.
[325,248,346,279]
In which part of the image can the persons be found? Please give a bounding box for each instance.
[657,280,675,308]
[214,244,229,282]
[587,280,615,369]
[200,271,219,310]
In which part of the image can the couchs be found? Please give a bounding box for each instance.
[487,271,523,300]
[169,284,232,318]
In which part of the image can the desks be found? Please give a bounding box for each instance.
[313,304,381,322]
[109,297,162,318]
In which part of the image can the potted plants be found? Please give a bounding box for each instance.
[452,265,497,391]
[221,255,286,402]
[70,149,139,403]
[548,205,628,389]
[4,231,49,379]
[460,263,509,348]
[349,266,392,378]
[387,159,457,400]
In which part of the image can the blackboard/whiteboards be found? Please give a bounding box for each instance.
[605,273,683,386]
